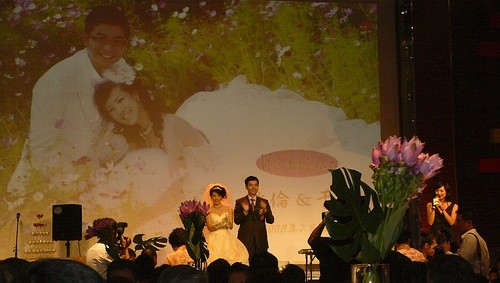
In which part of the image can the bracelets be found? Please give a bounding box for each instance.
[432,206,435,209]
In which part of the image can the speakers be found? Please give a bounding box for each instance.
[52,204,82,240]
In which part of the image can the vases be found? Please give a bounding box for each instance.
[350,263,391,283]
[195,259,207,270]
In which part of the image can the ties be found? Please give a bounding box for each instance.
[251,199,255,212]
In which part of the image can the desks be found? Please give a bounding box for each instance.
[299,249,318,281]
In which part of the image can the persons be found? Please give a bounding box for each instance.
[385,207,490,283]
[308,220,352,283]
[234,176,274,262]
[0,249,305,283]
[426,181,459,252]
[85,218,131,279]
[203,184,250,267]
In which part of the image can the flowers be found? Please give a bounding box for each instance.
[321,135,444,283]
[83,217,167,260]
[103,65,135,84]
[170,200,209,262]
[255,207,264,221]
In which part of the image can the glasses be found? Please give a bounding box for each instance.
[92,35,127,47]
